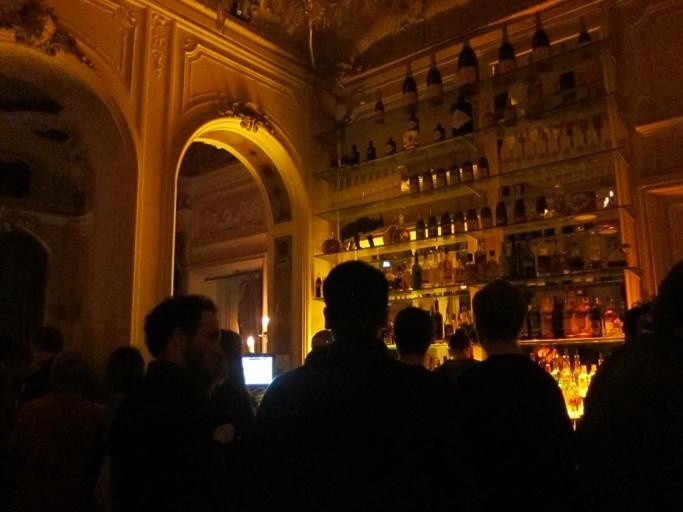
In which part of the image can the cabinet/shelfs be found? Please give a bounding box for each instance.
[310,0,646,351]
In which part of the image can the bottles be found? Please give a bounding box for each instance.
[461,145,473,182]
[495,187,508,225]
[480,190,492,229]
[577,18,593,44]
[409,165,419,195]
[330,155,338,166]
[422,160,434,190]
[392,214,410,242]
[482,104,494,128]
[375,89,384,118]
[557,56,576,102]
[450,152,461,185]
[449,90,474,135]
[508,118,614,166]
[401,61,418,105]
[426,351,450,370]
[426,55,443,97]
[453,195,466,233]
[510,184,624,338]
[416,208,425,240]
[407,111,421,147]
[385,138,396,155]
[522,65,546,117]
[433,122,445,140]
[434,157,447,187]
[379,246,502,291]
[534,10,551,61]
[425,294,471,342]
[477,142,490,179]
[530,346,607,418]
[497,26,516,73]
[466,194,478,231]
[364,140,376,160]
[427,207,438,238]
[384,216,398,244]
[502,96,515,120]
[458,38,479,85]
[348,146,360,164]
[441,202,450,236]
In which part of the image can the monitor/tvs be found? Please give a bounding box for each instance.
[240,354,274,387]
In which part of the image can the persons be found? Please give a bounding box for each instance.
[2,259,683,512]
